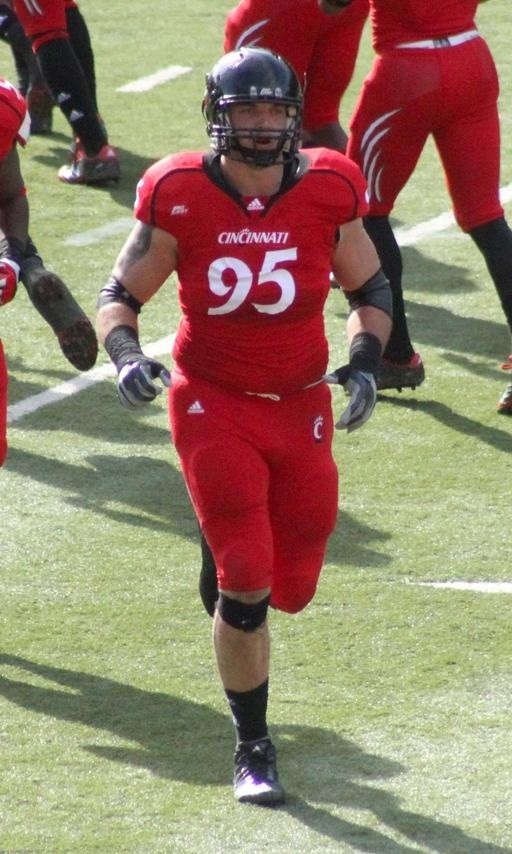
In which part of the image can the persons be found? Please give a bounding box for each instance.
[224,0,374,290]
[1,76,30,470]
[1,0,124,184]
[94,41,396,808]
[321,1,511,414]
[0,231,99,372]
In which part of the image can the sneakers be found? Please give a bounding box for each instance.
[59,144,121,185]
[374,354,425,390]
[233,737,283,804]
[497,387,512,413]
[27,270,97,370]
[200,535,219,616]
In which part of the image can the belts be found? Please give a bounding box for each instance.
[395,30,479,48]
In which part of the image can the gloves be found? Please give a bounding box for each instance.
[116,355,171,409]
[322,353,376,433]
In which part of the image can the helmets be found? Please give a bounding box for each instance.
[202,46,302,167]
